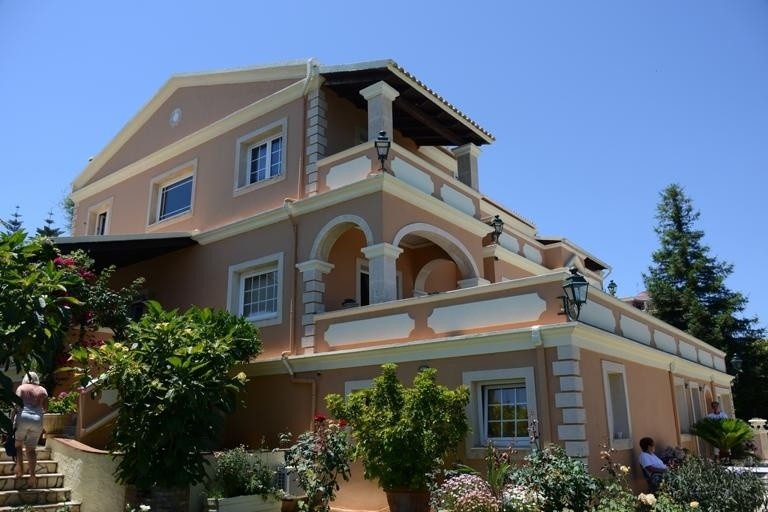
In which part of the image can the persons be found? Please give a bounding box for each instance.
[10,372,49,487]
[707,401,733,466]
[638,438,669,486]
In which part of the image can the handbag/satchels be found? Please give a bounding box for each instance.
[5,437,15,457]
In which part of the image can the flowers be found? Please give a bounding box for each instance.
[48,391,80,414]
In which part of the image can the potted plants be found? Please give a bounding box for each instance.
[323,351,473,510]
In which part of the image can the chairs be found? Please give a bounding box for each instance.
[639,463,664,492]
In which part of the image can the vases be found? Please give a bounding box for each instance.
[43,413,64,434]
